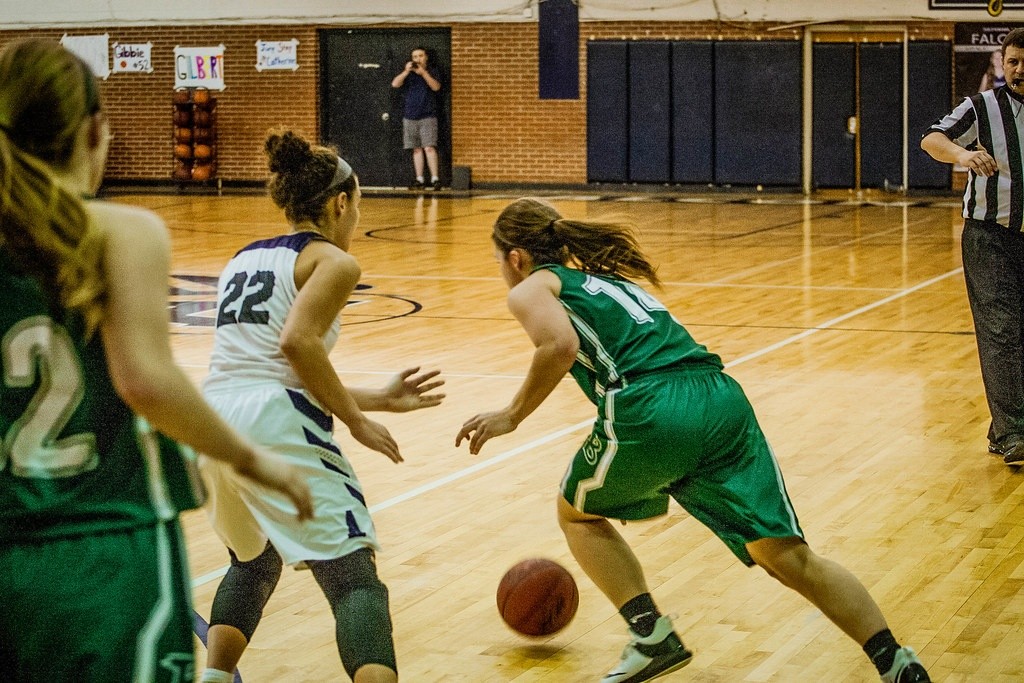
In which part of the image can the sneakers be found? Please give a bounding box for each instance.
[986,436,1024,466]
[408,179,425,190]
[878,645,931,683]
[598,613,694,683]
[431,179,444,191]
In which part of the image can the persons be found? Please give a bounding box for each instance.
[453,199,933,683]
[390,46,442,190]
[921,28,1023,467]
[978,50,1006,94]
[0,34,314,683]
[194,127,447,683]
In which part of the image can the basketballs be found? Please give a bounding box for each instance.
[496,558,579,638]
[171,85,215,184]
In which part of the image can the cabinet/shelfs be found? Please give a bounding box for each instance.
[172,87,221,188]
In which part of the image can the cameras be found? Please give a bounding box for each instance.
[411,62,421,68]
